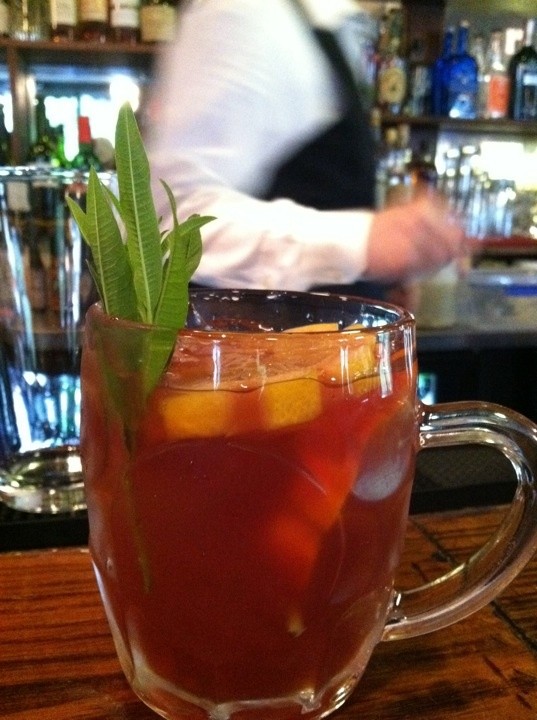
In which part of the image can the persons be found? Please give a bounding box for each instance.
[119,0,466,330]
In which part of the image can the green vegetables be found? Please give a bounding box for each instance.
[66,101,220,592]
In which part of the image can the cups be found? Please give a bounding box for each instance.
[0,161,119,516]
[81,289,537,720]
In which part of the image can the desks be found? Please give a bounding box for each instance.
[0,505,537,720]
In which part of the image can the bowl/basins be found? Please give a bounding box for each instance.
[463,270,536,334]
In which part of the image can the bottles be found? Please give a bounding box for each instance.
[0,0,184,43]
[0,105,34,221]
[369,11,537,242]
[31,105,70,219]
[69,116,103,215]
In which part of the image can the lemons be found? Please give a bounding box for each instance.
[158,322,378,437]
